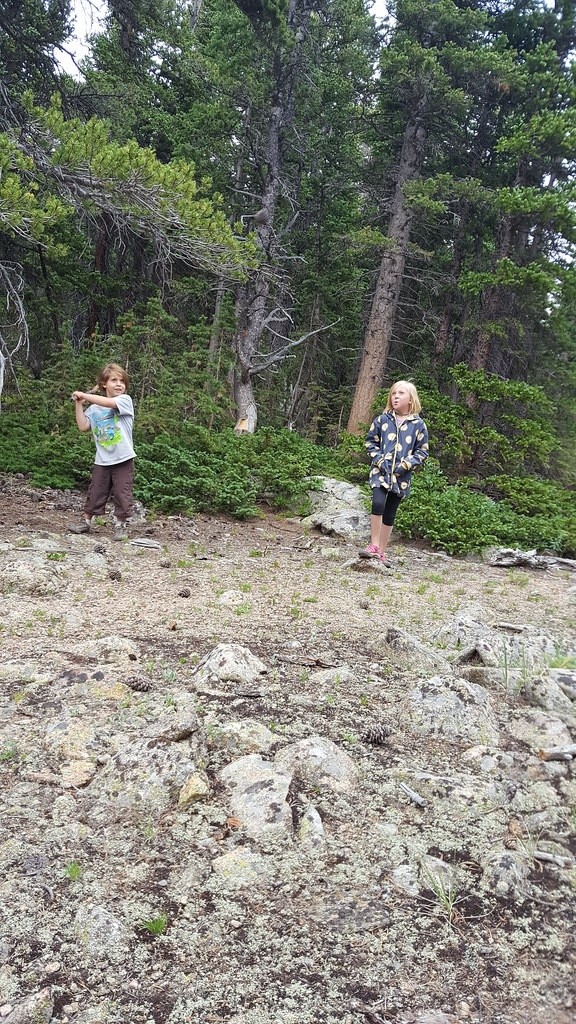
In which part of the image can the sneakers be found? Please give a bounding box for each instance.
[377,552,390,568]
[68,521,91,534]
[359,544,380,559]
[113,522,128,541]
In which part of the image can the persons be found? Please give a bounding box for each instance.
[356,380,429,563]
[67,363,138,541]
